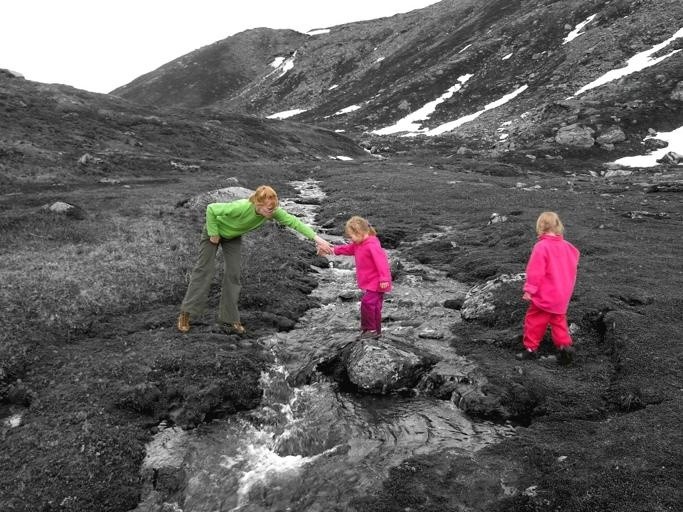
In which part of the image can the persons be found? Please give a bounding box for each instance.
[515,211,580,365]
[318,215,392,338]
[176,185,333,334]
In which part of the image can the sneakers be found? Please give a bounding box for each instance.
[232,322,245,333]
[560,346,571,365]
[178,312,189,332]
[356,330,377,340]
[516,350,538,360]
